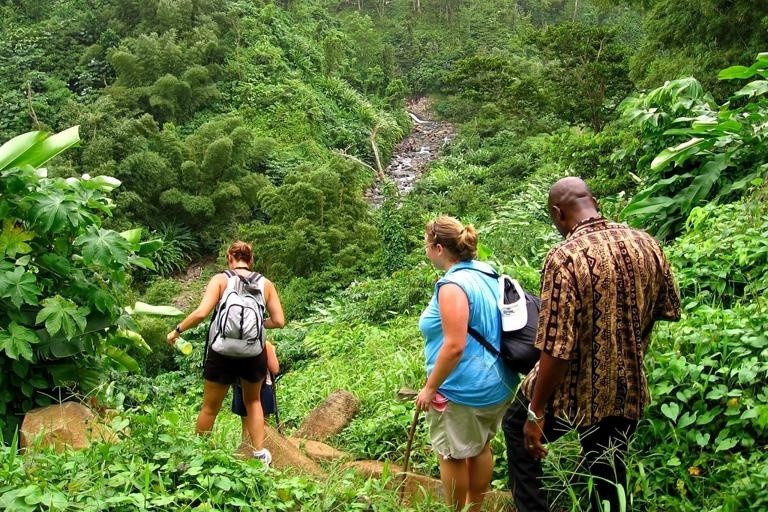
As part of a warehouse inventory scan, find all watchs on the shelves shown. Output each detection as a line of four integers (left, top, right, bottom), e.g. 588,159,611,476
523,402,545,424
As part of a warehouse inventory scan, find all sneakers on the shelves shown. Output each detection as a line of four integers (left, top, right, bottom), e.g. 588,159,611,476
254,447,273,476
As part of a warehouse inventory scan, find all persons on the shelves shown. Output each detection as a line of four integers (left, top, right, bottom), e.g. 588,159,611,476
231,338,282,444
501,175,683,512
165,240,289,469
415,214,522,512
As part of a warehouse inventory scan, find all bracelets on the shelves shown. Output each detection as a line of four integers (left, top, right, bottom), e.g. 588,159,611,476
175,323,182,333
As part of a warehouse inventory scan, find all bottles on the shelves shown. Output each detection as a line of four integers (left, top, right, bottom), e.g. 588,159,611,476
174,336,193,355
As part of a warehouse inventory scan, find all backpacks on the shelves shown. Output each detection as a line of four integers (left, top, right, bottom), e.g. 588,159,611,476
450,261,541,376
208,269,267,358
